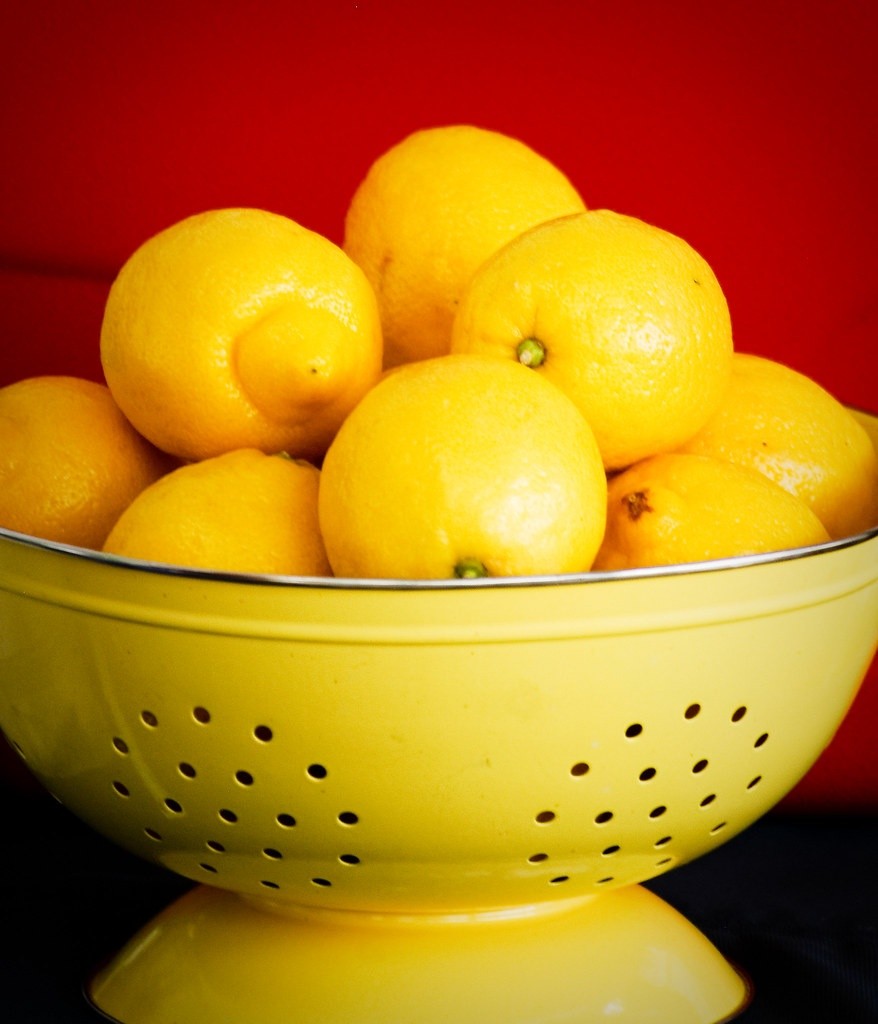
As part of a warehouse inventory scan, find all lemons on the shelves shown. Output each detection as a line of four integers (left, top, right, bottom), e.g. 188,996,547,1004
0,124,878,583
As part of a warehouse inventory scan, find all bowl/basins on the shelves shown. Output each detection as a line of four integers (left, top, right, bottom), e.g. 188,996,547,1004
0,411,878,1024
